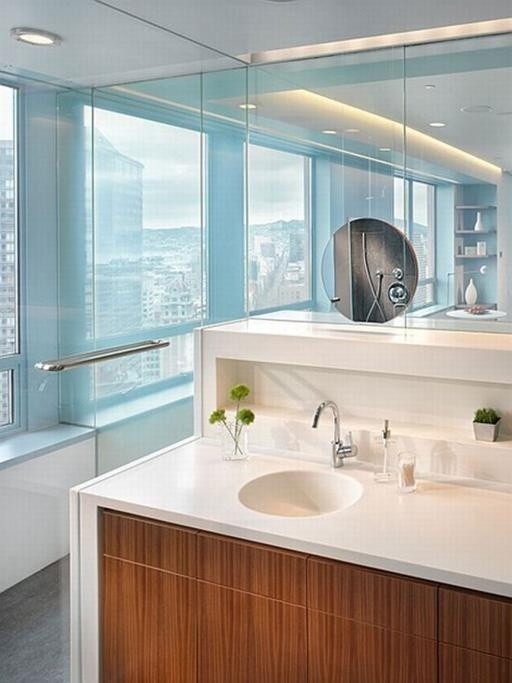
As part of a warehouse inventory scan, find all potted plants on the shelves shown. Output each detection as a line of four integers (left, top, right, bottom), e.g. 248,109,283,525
472,408,502,442
208,383,257,461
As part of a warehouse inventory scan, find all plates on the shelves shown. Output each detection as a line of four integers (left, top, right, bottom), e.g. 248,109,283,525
446,309,508,320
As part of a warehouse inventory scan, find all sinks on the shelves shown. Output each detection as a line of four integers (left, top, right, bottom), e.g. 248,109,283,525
236,467,364,520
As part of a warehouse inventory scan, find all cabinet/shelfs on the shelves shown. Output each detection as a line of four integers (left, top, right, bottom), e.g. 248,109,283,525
95,510,511,681
454,201,498,310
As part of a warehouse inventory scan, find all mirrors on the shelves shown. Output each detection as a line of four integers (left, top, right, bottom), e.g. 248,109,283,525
317,214,421,328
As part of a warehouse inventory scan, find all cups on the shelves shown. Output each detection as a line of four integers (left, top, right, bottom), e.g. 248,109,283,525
477,240,487,255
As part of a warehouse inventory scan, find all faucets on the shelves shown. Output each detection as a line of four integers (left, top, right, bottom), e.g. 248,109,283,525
311,401,360,468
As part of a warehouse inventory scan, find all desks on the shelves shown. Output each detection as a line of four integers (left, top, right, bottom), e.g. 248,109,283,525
445,307,507,321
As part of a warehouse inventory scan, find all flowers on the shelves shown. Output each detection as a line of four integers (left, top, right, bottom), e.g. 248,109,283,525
464,305,488,313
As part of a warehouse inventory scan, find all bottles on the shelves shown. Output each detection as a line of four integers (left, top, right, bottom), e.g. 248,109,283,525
474,211,485,231
465,278,477,307
396,449,417,493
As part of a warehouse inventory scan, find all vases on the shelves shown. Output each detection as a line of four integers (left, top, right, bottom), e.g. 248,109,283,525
474,210,486,231
464,278,479,304
